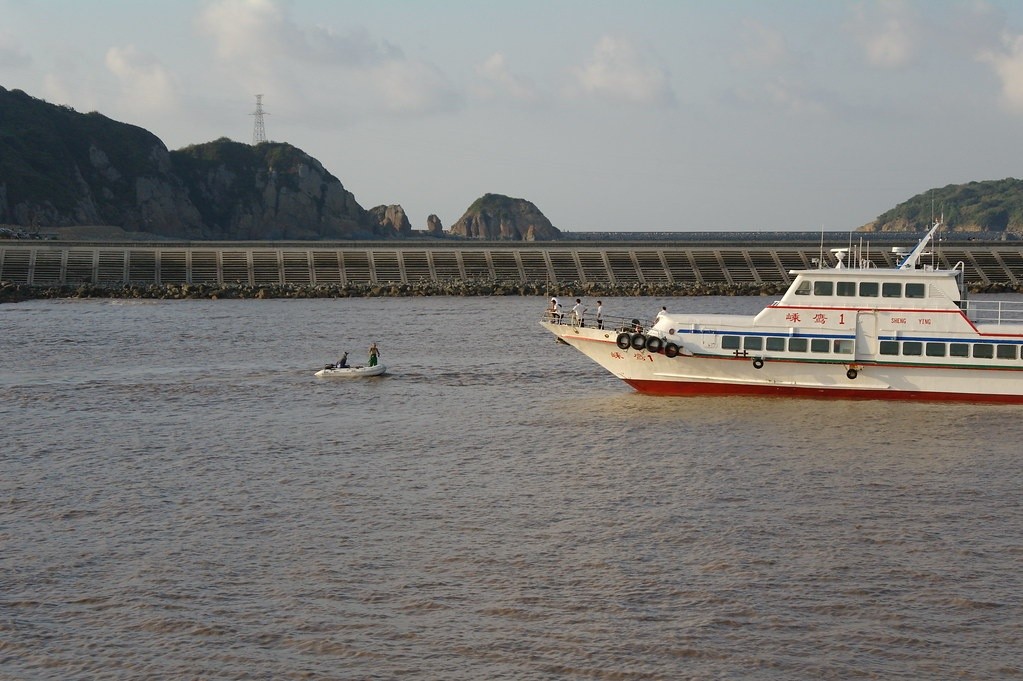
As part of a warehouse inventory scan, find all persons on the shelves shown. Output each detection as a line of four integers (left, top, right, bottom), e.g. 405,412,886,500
548,291,565,327
368,341,381,366
334,350,351,368
597,300,605,330
653,306,668,322
567,298,588,326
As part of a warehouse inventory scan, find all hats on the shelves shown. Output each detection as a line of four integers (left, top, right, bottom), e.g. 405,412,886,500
343,351,349,355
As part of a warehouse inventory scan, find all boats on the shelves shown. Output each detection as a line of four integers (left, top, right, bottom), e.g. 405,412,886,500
541,221,1023,406
315,364,385,376
